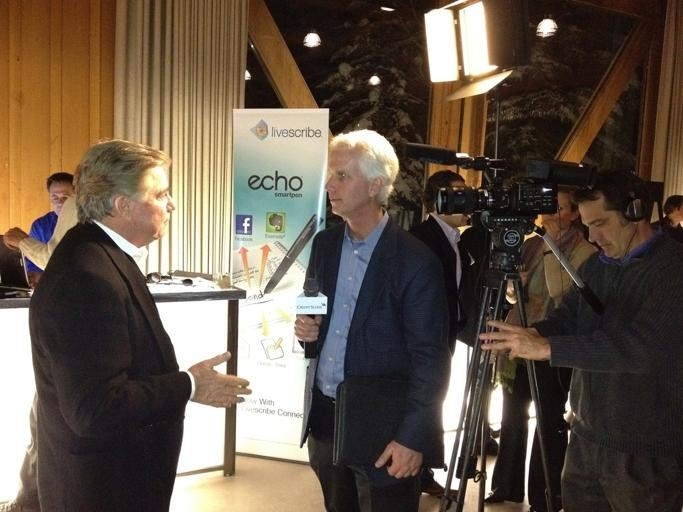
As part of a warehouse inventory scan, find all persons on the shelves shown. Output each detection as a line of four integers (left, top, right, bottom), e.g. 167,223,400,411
4,140,251,511
22,172,77,291
290,126,451,512
412,170,682,512
3,165,79,271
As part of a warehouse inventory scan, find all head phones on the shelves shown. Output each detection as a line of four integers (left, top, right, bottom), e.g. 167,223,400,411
619,168,643,222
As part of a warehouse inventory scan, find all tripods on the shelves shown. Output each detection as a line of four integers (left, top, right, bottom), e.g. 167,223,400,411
437,266,558,512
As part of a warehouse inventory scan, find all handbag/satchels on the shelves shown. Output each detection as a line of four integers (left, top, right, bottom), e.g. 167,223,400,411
332,378,446,467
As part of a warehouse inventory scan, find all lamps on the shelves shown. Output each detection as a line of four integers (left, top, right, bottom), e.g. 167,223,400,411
423,0,530,102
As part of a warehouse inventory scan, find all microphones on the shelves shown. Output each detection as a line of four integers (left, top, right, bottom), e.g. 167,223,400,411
296,280,328,359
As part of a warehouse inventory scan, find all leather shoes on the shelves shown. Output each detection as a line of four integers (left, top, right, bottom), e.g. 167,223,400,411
484,489,525,503
